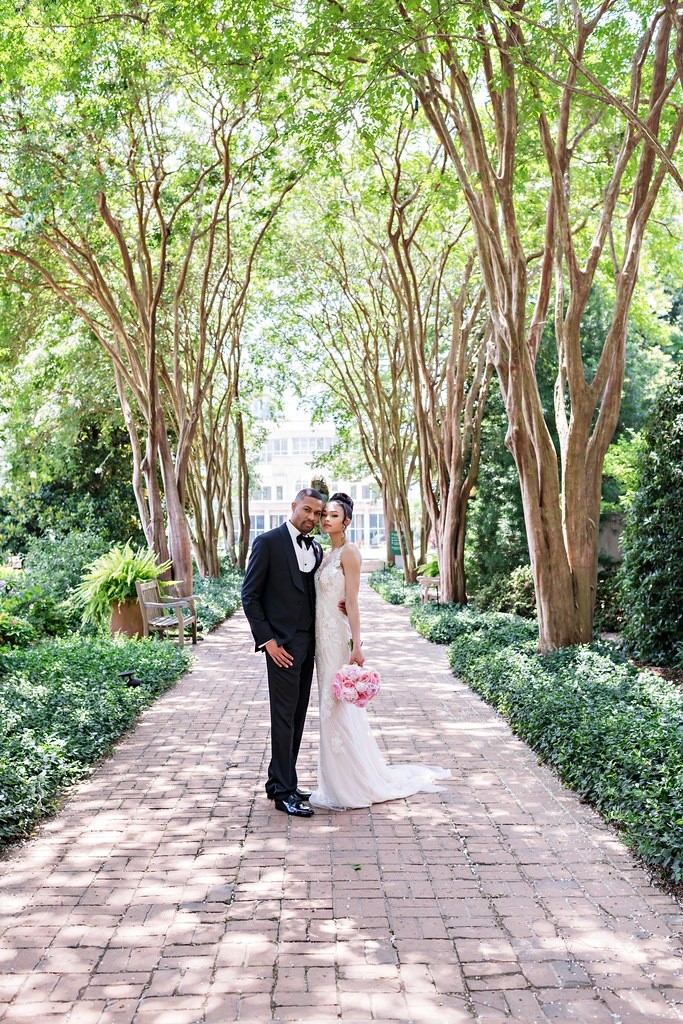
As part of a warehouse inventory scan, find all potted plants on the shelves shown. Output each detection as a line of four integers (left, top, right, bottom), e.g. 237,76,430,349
63,536,183,641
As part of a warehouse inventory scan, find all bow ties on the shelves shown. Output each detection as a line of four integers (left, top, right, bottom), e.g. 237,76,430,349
297,534,314,550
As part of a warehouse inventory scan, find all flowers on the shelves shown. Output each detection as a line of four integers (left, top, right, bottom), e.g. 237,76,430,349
332,638,381,709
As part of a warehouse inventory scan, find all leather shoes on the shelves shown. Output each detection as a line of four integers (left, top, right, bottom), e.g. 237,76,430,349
276,794,314,815
268,788,311,800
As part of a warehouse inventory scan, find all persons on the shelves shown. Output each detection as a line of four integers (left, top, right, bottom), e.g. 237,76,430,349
241,488,348,817
307,493,450,809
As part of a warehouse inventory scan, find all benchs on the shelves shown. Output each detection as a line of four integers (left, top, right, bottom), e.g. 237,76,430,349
416,576,439,605
134,579,202,645
